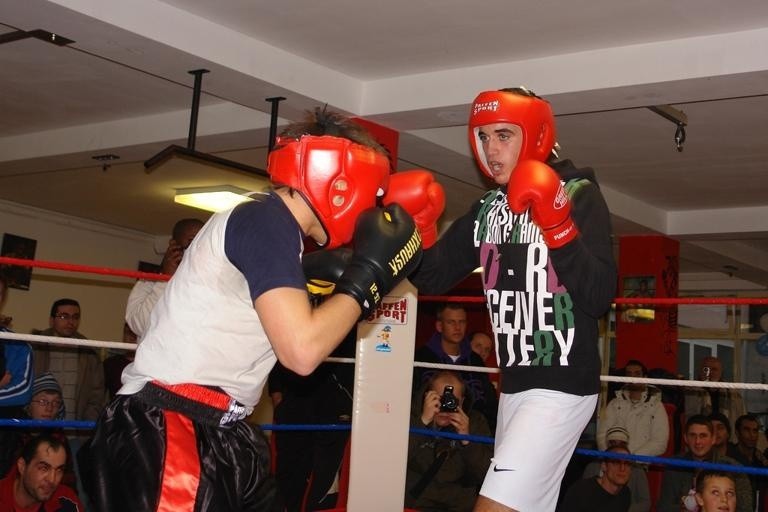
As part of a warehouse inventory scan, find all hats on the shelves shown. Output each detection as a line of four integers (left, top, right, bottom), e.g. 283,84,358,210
31,370,62,397
605,424,628,444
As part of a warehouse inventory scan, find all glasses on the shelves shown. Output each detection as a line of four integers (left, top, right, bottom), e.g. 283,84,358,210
55,313,70,320
32,398,59,407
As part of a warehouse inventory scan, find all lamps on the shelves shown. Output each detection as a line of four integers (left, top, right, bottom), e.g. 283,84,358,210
174,184,254,213
144,67,284,193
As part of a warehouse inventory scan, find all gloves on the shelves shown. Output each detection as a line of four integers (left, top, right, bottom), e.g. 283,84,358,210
381,170,446,251
507,158,579,251
303,247,353,297
332,203,423,322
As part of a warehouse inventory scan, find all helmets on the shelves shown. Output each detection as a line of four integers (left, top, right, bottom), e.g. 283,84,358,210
469,85,555,181
266,129,390,249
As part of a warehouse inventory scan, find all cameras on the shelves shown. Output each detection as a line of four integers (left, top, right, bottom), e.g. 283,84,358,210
438,384,459,411
